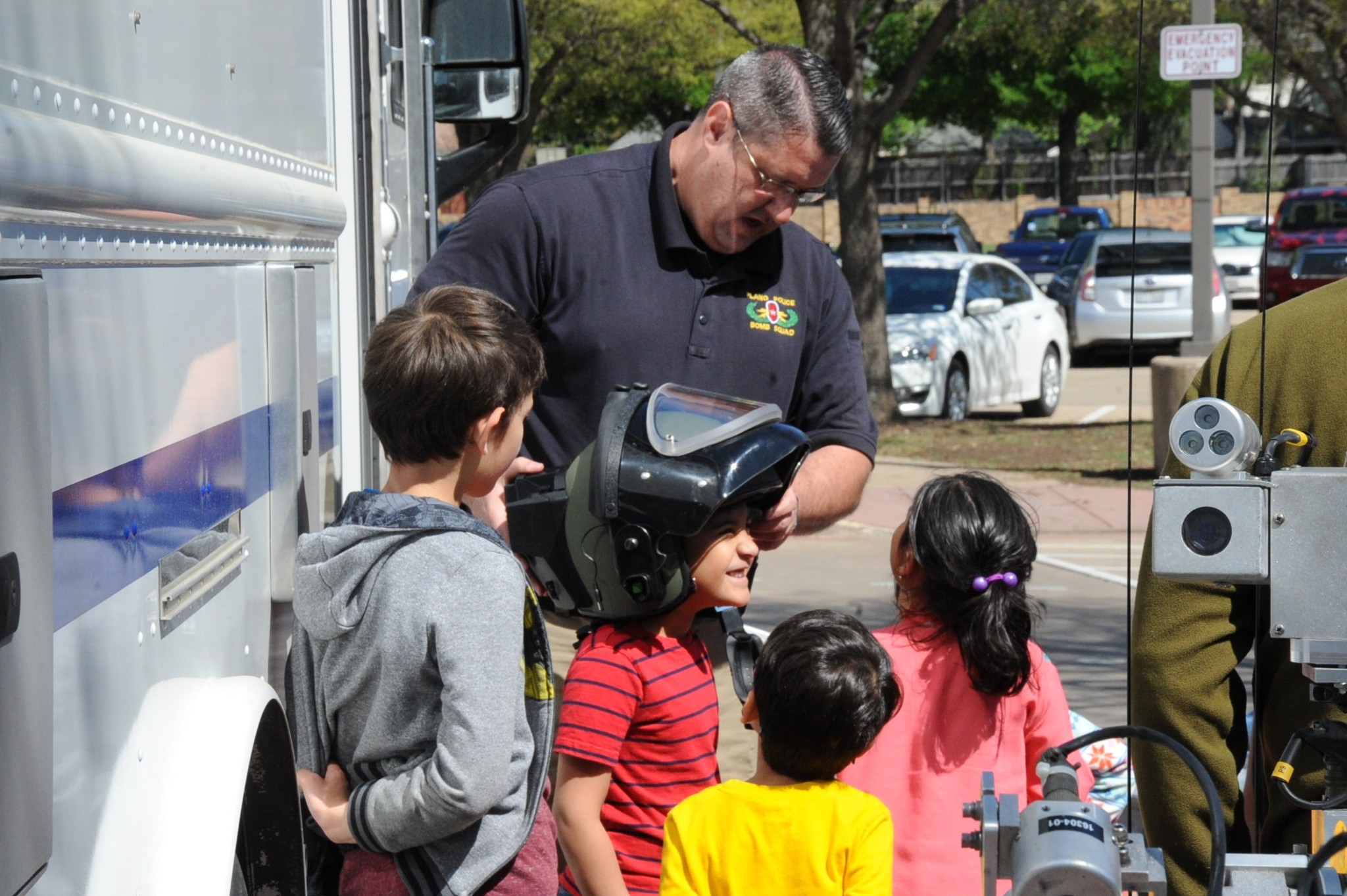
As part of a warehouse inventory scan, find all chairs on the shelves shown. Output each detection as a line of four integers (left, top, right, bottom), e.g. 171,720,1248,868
1295,205,1317,223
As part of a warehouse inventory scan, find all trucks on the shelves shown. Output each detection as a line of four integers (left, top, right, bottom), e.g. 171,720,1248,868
0,1,531,896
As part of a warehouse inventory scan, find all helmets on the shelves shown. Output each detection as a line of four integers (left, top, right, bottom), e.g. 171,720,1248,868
507,378,813,621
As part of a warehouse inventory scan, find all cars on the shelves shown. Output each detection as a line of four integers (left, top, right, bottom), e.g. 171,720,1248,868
836,251,1072,420
1209,214,1274,302
872,212,985,255
1046,226,1231,359
1246,152,1347,311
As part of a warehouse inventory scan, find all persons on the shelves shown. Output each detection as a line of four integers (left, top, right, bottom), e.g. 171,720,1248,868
52,337,270,538
659,609,904,896
404,42,878,681
553,382,813,896
290,284,559,896
834,472,1096,896
1130,276,1347,895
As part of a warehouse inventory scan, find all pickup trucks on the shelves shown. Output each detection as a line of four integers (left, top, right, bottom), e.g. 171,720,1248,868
995,205,1118,289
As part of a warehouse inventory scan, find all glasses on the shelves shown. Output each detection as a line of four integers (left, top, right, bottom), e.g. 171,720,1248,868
728,98,827,207
